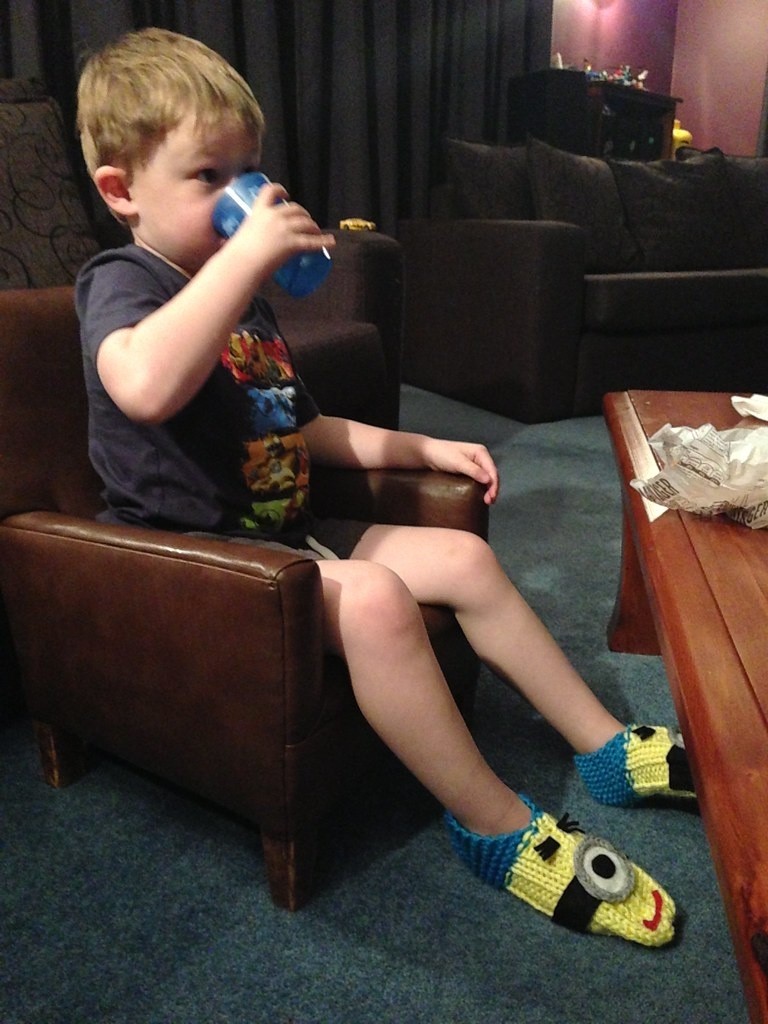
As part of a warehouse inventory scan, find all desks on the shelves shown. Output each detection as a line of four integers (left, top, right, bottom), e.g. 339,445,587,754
507,70,684,163
601,390,768,1024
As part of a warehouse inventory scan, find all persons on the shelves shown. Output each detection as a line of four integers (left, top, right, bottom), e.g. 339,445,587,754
70,25,707,943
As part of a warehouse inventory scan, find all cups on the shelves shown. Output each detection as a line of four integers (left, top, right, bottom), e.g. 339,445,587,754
211,170,331,300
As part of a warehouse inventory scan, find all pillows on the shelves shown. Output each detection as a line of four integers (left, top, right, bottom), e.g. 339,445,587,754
0,93,104,290
429,136,768,273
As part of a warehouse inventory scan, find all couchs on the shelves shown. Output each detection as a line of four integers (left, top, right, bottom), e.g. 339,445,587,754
397,136,768,427
0,74,407,431
0,284,489,911
